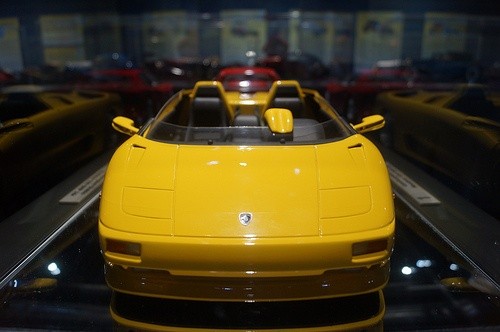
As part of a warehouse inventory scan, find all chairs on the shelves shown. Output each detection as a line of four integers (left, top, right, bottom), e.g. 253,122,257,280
186,97,227,142
273,98,325,144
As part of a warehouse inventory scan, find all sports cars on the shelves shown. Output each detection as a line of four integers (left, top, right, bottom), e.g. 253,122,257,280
97,80,397,303
0,51,500,178
